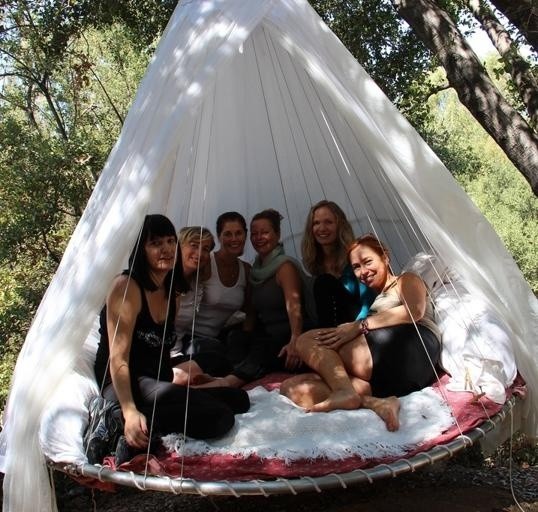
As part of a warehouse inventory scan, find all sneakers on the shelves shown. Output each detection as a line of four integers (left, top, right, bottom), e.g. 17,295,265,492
82,396,142,467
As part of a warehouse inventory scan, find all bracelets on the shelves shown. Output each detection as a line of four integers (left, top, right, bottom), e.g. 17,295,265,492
360,317,369,335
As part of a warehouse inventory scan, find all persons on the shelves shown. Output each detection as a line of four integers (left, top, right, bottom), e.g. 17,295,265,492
169,227,235,388
281,233,443,432
299,200,371,326
220,208,314,374
85,214,250,467
191,212,256,388
